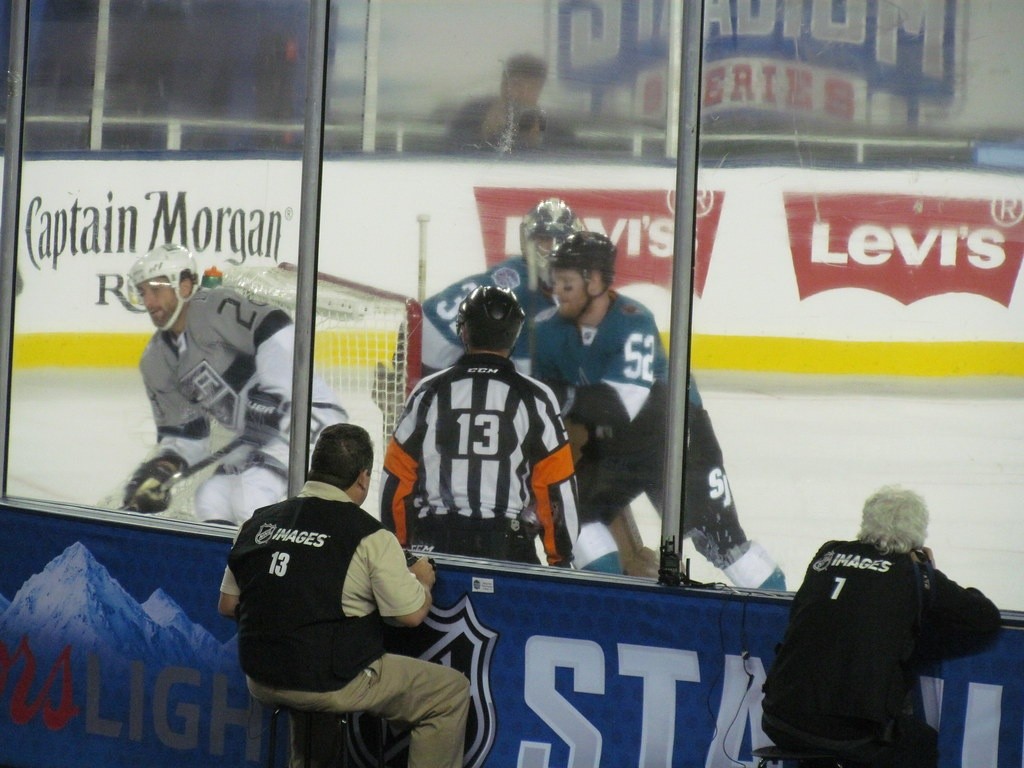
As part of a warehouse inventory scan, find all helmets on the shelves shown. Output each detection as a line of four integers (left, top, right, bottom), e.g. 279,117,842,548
547,230,617,286
128,243,202,303
525,198,575,240
455,284,525,350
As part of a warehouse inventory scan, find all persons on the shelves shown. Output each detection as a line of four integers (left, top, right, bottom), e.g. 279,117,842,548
516,229,786,593
447,54,573,152
378,284,581,568
121,244,350,527
217,425,471,768
392,196,583,394
760,486,1001,768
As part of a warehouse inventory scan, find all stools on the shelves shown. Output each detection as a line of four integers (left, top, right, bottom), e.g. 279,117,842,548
750,746,853,768
269,705,386,768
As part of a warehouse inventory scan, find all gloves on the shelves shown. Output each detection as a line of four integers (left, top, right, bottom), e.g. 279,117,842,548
125,448,191,513
243,384,284,445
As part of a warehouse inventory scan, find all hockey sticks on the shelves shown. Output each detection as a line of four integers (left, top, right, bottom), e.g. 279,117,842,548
117,432,246,512
